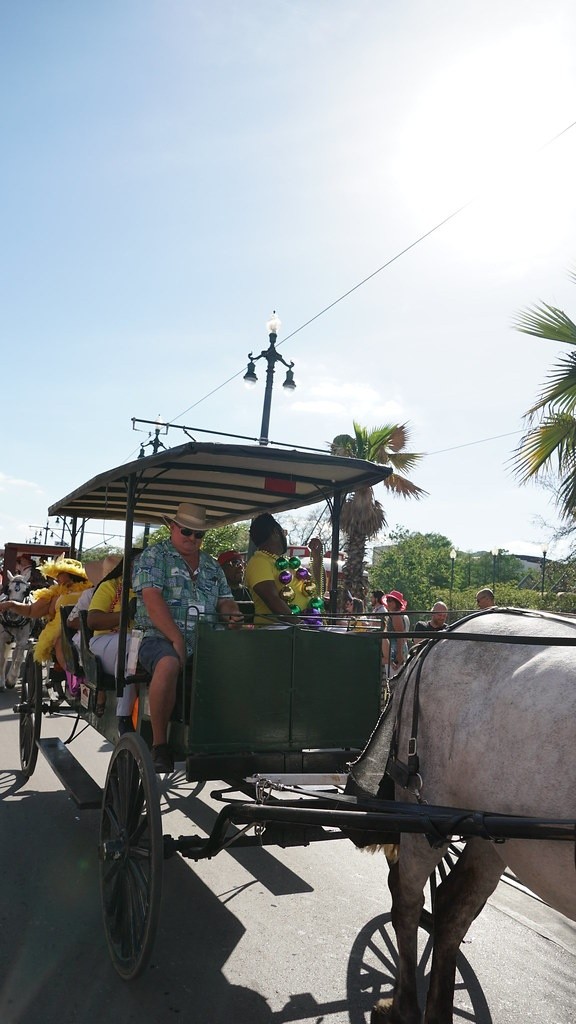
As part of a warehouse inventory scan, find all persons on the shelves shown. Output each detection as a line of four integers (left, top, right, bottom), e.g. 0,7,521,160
0,553,57,643
410,601,450,657
476,588,497,611
215,548,258,625
380,590,410,697
85,544,147,746
348,598,371,632
126,499,245,779
0,557,96,696
336,600,354,627
368,589,388,706
64,554,126,703
242,512,327,626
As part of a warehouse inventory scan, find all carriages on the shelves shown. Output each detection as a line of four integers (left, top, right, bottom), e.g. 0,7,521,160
0,542,77,692
11,417,576,1024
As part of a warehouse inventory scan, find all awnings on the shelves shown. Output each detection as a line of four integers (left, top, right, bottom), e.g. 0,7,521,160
44,439,395,542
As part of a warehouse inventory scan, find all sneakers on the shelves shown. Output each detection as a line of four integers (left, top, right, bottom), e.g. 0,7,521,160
118,716,136,740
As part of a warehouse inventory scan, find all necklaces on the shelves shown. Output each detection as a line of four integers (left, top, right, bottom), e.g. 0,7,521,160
108,578,122,634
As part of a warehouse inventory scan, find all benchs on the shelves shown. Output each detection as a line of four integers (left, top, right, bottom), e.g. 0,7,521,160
59,603,255,691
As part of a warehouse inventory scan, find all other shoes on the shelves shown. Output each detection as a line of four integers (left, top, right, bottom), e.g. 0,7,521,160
94,701,105,717
151,743,173,773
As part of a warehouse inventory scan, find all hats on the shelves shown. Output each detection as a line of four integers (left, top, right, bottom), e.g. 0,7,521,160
323,591,331,600
218,551,243,566
34,552,88,581
161,503,216,536
16,553,33,566
381,590,407,612
85,556,121,588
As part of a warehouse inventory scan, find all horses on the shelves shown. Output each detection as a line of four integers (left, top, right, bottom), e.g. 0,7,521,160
355,605,576,1024
0,568,37,694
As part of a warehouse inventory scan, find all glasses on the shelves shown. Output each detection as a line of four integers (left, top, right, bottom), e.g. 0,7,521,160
175,525,205,539
279,530,287,536
386,599,395,603
477,597,487,603
227,559,248,570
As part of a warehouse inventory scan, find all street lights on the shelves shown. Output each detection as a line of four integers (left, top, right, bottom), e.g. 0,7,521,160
243,309,297,561
449,548,457,623
541,542,548,601
490,546,498,593
25,514,74,545
137,429,174,547
